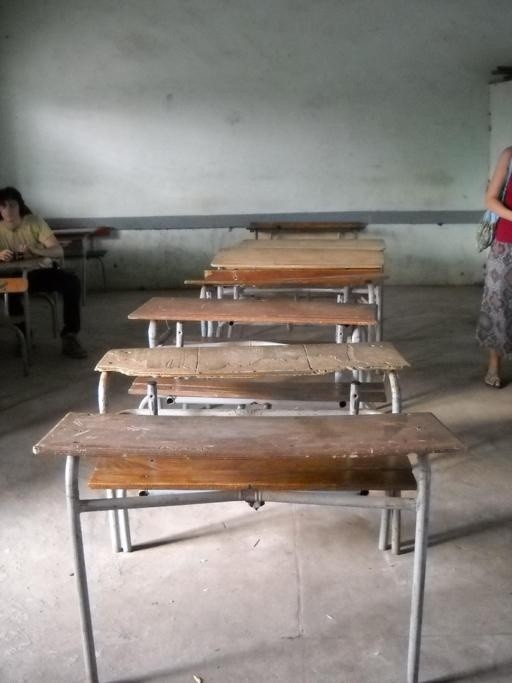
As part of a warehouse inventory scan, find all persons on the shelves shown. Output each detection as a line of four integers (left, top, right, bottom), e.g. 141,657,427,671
1,184,90,359
472,144,512,387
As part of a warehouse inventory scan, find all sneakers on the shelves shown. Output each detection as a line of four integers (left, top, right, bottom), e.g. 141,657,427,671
15,334,36,357
60,335,89,359
484,365,505,388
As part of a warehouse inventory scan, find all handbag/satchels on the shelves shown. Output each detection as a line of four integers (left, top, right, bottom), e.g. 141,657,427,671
475,208,499,254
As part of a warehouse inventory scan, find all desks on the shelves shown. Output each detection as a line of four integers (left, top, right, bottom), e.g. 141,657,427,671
93,342,411,554
32,412,469,682
127,222,388,411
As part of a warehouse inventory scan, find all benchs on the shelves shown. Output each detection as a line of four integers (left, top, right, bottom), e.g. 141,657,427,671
3,226,115,373
87,453,416,554
